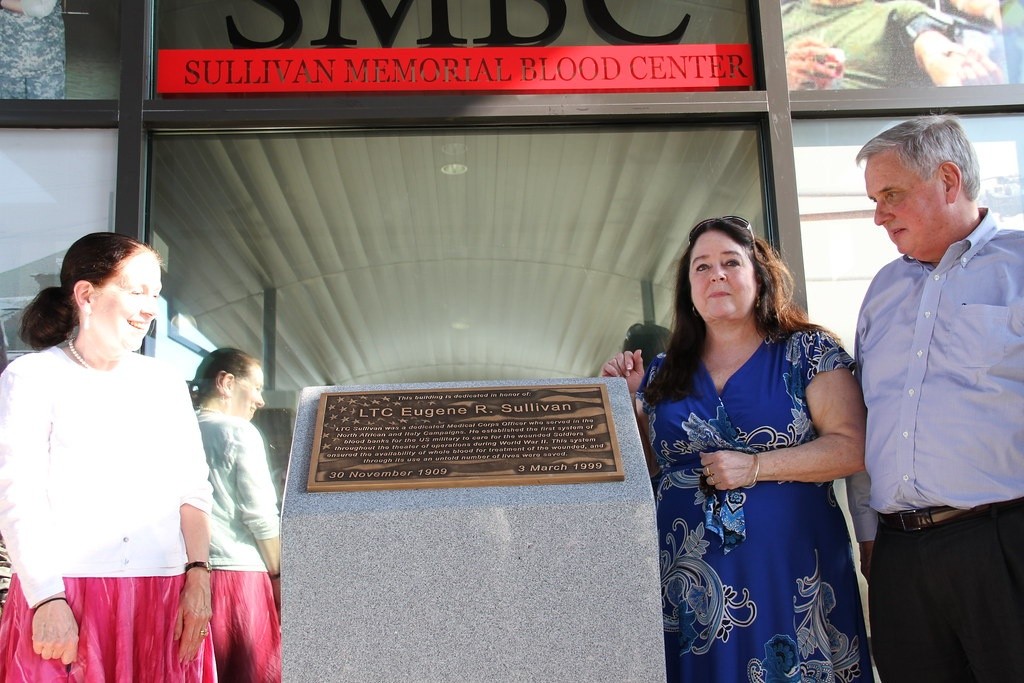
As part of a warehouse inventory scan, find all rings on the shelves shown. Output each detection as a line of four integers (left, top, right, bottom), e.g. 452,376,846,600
710,476,717,484
706,465,712,476
201,629,207,636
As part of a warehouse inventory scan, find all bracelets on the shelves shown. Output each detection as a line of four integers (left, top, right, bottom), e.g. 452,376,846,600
270,573,280,579
748,453,759,487
34,597,68,615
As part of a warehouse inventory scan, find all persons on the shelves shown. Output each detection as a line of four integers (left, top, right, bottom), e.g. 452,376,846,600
780,0,1001,91
0,231,219,683
188,346,281,683
601,217,875,683
623,323,676,373
846,118,1024,683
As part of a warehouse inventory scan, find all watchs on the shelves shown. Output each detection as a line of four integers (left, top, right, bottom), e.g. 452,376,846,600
185,561,212,573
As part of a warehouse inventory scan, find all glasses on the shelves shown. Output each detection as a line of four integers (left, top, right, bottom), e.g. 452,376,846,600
688,215,756,249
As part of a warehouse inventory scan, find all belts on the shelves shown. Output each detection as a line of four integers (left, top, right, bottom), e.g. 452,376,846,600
877,501,996,532
